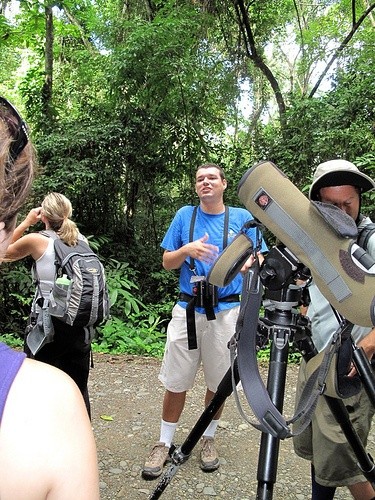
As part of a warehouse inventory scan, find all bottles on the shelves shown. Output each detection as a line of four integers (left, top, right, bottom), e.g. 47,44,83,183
56,274,72,291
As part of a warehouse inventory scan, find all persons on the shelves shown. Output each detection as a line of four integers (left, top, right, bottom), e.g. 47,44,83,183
5,192,91,418
0,96,100,500
240,160,375,500
142,163,269,476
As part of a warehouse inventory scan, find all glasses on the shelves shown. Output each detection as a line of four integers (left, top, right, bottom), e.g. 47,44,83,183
0,97,29,174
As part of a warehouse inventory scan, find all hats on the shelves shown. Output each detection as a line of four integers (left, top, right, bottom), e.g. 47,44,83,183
309,160,375,200
27,309,54,356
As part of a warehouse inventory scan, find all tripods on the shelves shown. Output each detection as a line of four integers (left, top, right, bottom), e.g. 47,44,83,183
150,245,375,500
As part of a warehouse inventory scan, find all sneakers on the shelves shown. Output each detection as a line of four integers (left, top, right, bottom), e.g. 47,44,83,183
143,442,170,477
200,436,219,471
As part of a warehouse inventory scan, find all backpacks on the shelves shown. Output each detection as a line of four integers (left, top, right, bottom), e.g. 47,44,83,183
42,230,109,327
